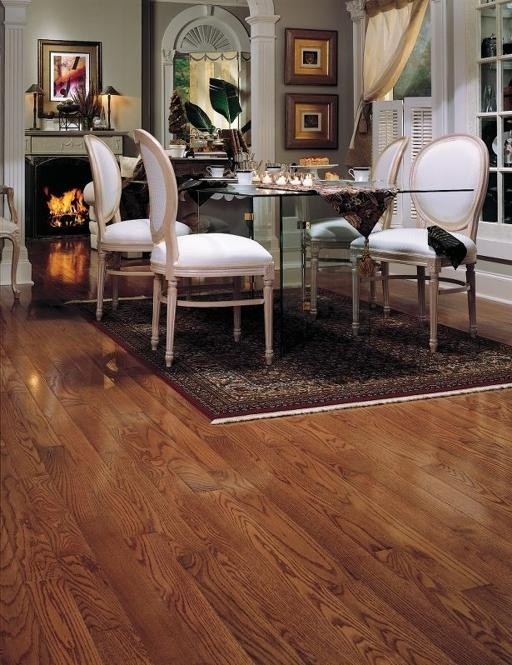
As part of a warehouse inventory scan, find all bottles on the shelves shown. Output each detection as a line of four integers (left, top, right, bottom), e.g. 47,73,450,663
482,80,496,111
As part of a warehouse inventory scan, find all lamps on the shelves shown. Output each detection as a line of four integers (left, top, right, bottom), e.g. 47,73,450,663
99,85,120,129
26,83,46,129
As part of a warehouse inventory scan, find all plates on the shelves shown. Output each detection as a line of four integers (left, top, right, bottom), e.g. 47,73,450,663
228,183,257,187
320,179,343,182
203,175,230,179
345,179,374,183
289,163,339,169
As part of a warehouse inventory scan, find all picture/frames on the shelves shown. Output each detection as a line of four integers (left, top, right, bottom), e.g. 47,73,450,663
284,27,338,86
38,38,102,119
285,92,340,149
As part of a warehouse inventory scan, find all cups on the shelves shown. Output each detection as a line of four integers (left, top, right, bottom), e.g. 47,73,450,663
206,165,226,176
246,160,314,187
349,166,371,182
236,170,253,184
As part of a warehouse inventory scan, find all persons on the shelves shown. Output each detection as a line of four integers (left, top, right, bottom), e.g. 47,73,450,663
481,82,512,224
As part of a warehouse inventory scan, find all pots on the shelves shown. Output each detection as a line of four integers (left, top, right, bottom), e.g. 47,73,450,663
481,32,503,57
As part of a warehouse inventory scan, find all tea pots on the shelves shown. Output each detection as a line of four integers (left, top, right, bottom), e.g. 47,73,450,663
234,148,255,170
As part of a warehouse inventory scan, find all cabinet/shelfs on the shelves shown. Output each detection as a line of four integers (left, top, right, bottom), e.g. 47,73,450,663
453,0,512,262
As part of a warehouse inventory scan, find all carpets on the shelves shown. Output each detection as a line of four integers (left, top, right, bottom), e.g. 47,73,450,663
68,287,509,426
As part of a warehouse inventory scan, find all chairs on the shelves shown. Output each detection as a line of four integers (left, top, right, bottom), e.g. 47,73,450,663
82,146,185,251
133,128,275,366
350,135,489,354
0,186,23,300
300,137,410,319
85,134,191,321
